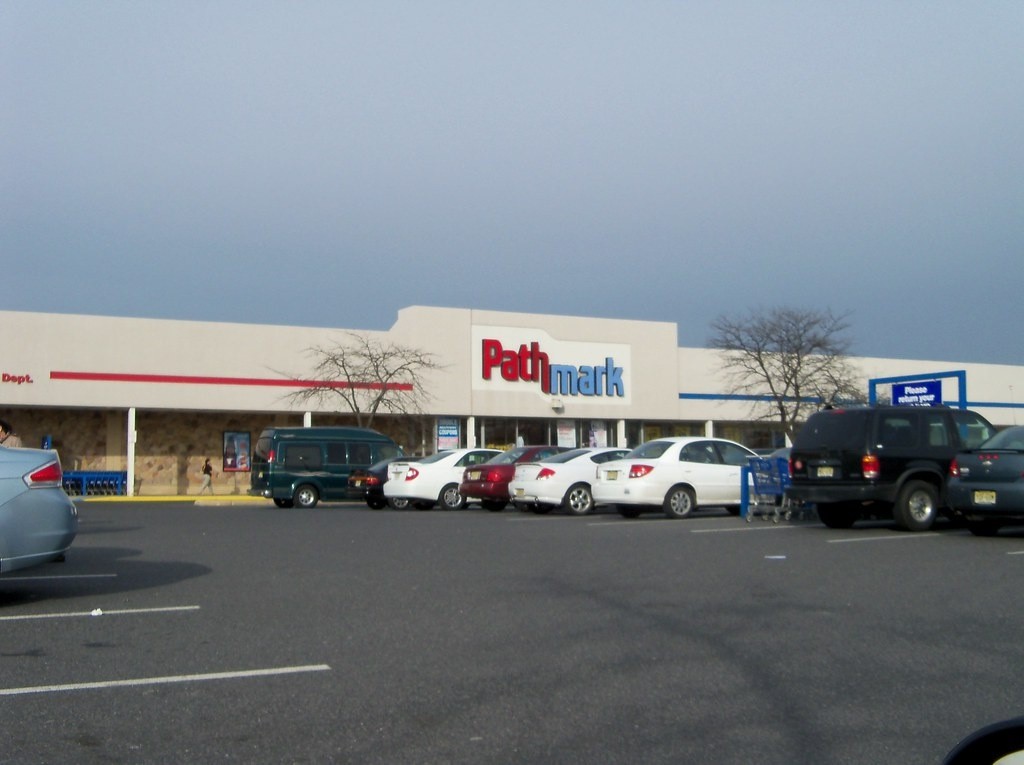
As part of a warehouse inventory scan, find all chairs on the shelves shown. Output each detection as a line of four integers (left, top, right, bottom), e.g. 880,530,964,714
885,423,920,446
688,450,719,463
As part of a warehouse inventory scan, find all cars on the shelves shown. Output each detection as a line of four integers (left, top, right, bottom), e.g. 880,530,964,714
591,436,790,521
0,444,78,573
381,449,506,512
347,456,425,512
507,447,635,516
947,424,1024,537
458,445,576,513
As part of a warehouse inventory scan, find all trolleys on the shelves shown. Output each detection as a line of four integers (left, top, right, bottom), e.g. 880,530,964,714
741,454,807,524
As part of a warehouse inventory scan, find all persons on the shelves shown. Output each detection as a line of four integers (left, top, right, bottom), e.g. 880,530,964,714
197,459,214,495
0,420,22,448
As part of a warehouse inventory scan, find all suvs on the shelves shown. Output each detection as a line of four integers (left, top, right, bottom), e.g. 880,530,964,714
785,405,999,534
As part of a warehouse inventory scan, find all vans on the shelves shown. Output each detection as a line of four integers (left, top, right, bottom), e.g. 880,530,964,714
244,426,412,511
